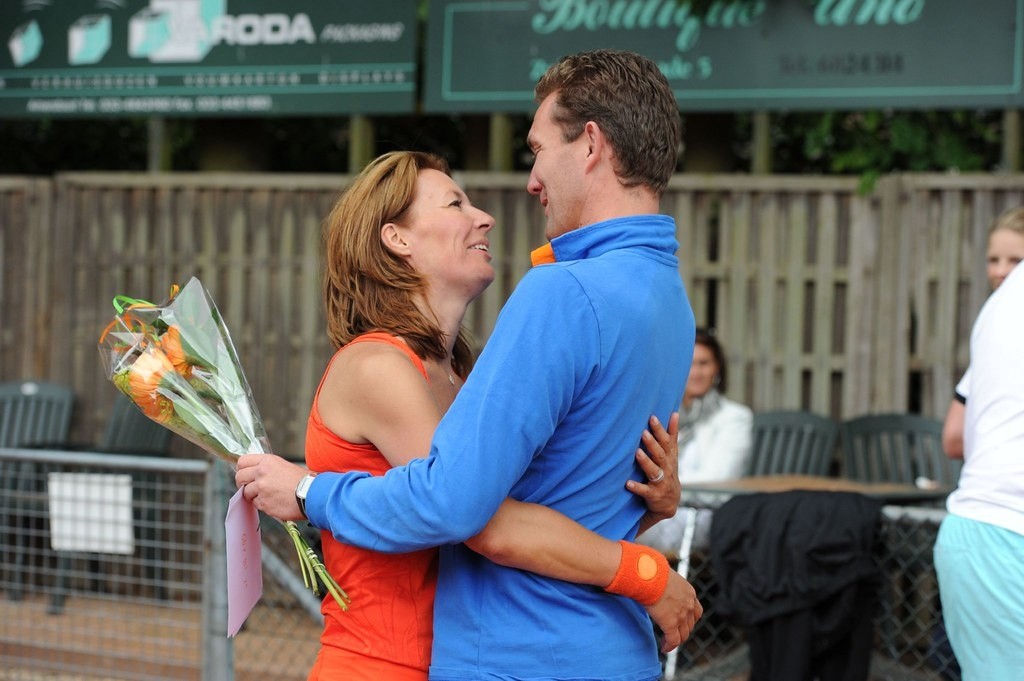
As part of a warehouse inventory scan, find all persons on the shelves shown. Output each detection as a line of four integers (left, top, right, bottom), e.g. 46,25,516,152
932,257,1024,681
634,325,753,555
236,51,695,679
304,149,703,681
984,203,1024,290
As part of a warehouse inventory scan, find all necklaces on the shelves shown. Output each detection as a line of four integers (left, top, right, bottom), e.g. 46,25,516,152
445,366,455,385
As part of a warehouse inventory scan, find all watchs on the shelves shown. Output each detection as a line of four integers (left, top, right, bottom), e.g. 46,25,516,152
295,471,320,527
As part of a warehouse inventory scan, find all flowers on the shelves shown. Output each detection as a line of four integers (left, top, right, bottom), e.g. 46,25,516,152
103,296,355,613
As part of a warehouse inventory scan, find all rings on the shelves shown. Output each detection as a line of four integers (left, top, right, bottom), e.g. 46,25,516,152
649,468,664,482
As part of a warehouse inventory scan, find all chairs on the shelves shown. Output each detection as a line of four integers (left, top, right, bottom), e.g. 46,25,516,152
51,393,174,613
743,407,843,479
0,385,81,591
837,412,960,492
